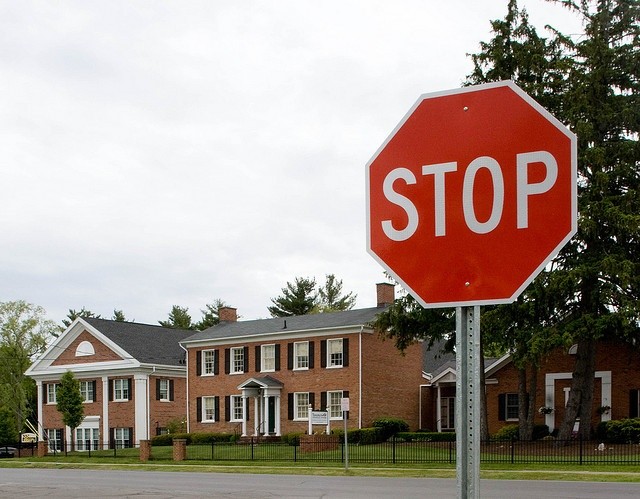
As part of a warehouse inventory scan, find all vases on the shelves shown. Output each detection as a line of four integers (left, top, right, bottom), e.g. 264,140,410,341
545,409,553,414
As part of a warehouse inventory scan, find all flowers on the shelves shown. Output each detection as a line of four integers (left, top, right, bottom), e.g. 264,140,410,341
539,407,555,416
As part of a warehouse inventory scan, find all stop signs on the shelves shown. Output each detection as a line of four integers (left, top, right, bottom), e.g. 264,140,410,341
365,79,577,310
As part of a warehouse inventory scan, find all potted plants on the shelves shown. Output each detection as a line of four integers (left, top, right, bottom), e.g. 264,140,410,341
597,406,610,415
